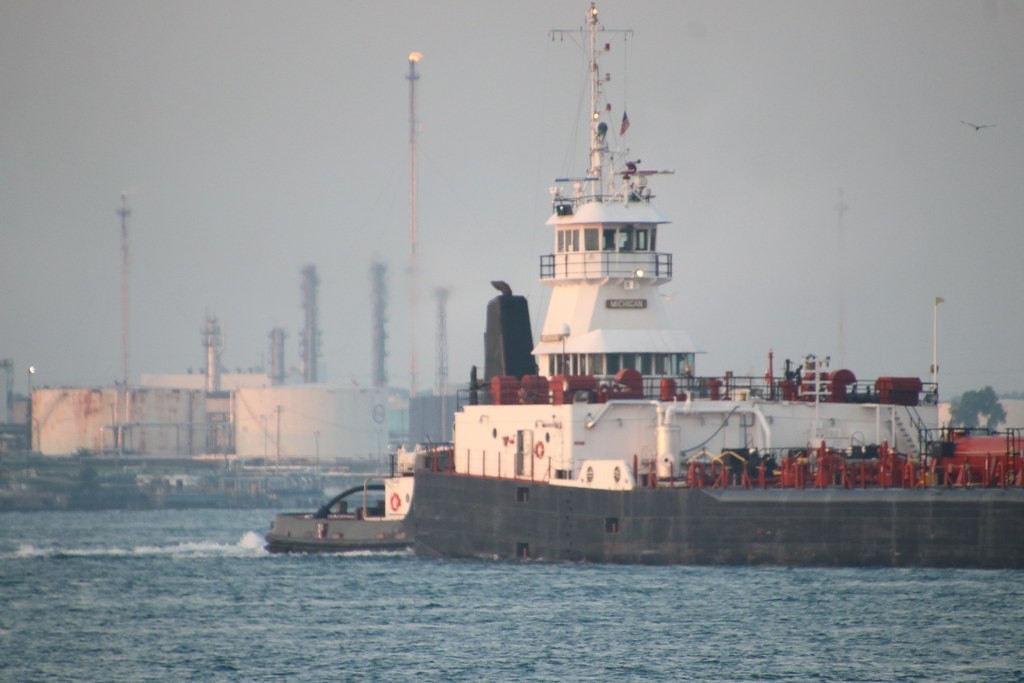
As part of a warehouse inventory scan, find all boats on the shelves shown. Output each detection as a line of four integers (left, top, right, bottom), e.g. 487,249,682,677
381,0,1023,574
262,475,415,552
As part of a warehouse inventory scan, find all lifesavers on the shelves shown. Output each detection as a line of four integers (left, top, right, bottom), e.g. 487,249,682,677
535,441,544,459
389,493,401,511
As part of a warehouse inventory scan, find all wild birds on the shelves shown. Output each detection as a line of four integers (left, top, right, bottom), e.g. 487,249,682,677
960,120,998,132
